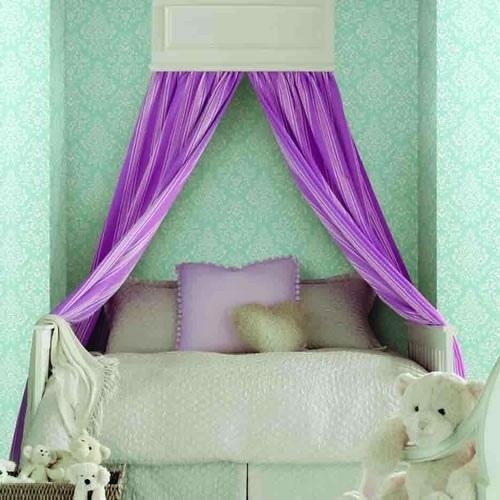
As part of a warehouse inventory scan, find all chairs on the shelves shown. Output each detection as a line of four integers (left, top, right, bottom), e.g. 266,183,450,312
384,344,500,499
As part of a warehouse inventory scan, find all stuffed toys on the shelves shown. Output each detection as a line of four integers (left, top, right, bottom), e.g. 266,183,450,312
68,463,111,500
18,443,63,483
50,429,111,482
0,459,17,483
363,369,485,500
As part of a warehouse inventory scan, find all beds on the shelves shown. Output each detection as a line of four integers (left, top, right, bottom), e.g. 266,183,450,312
32,0,454,498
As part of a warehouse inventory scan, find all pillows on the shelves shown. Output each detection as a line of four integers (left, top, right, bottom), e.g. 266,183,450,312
174,255,301,355
98,274,182,354
297,271,389,353
237,302,311,351
